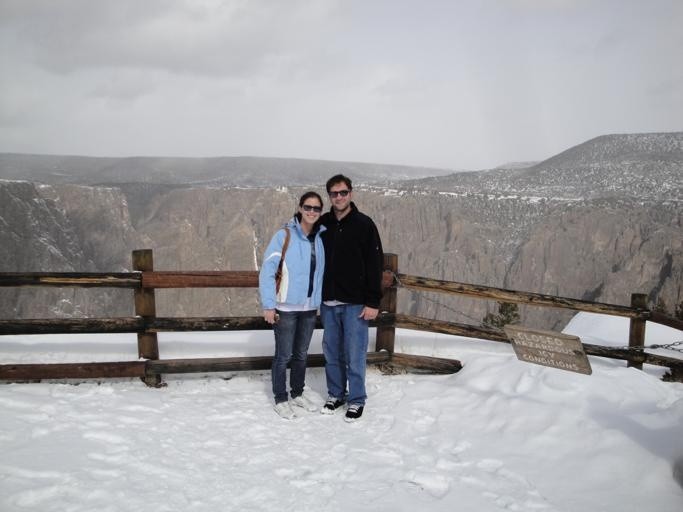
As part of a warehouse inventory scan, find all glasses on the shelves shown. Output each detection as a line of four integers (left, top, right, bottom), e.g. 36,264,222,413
302,205,322,213
328,190,350,198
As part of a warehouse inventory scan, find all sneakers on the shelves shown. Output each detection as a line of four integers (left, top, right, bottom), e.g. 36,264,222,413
273,401,297,421
290,394,317,412
345,403,363,418
323,394,347,410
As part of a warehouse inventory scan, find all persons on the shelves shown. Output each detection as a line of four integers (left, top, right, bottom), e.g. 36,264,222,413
313,175,385,421
259,191,328,420
